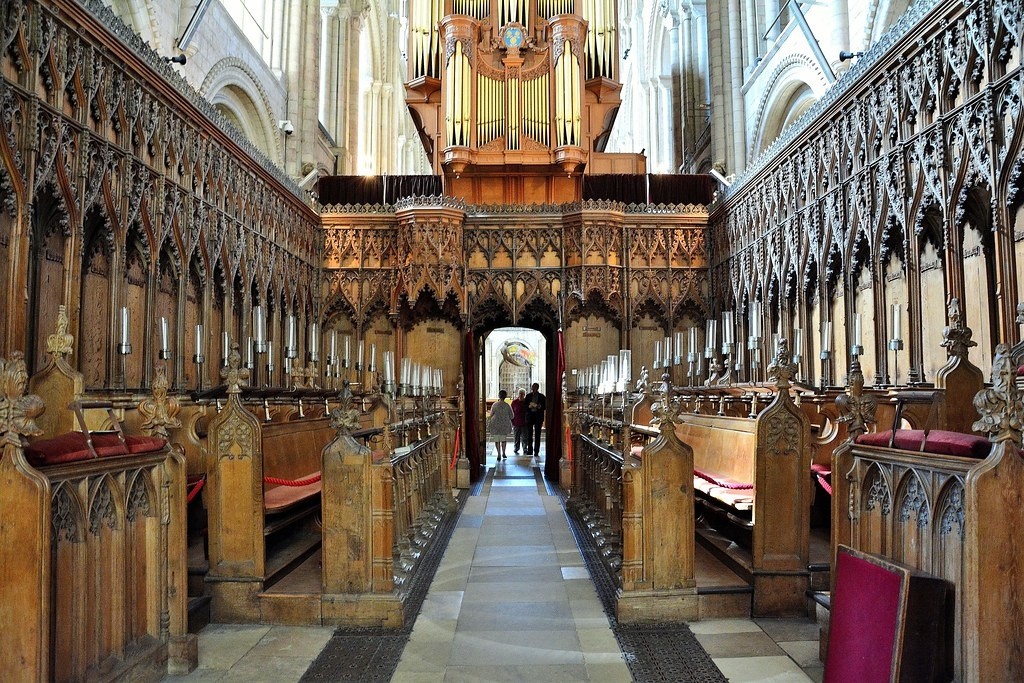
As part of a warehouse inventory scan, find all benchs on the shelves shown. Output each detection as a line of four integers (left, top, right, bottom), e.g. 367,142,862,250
633,383,895,577
164,398,405,586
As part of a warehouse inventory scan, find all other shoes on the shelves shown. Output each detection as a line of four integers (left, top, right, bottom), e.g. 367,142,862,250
534,452,538,456
513,450,518,452
502,455,507,458
524,450,527,453
497,455,501,461
527,451,533,455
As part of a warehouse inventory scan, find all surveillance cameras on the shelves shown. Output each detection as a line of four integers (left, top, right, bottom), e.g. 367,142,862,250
284,124,293,135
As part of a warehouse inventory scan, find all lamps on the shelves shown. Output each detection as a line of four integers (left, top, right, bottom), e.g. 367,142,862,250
292,166,320,189
839,50,863,63
708,169,736,188
165,53,187,65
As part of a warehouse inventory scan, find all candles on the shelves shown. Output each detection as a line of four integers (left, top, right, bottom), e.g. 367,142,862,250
576,299,832,395
195,324,200,355
122,306,128,344
894,303,901,340
162,316,168,351
221,305,444,389
855,313,863,347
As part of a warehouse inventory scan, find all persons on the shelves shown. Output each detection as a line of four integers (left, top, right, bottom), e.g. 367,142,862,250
489,390,514,461
511,389,528,453
524,383,545,456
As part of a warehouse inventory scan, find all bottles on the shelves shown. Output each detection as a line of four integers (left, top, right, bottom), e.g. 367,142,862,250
640,148,645,156
